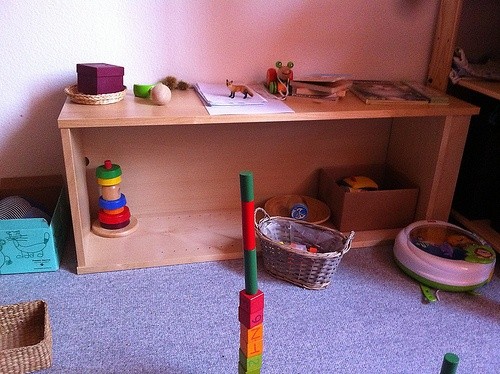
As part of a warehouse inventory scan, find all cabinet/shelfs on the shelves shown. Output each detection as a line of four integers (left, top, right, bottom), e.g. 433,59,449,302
451,75,500,253
57,81,481,276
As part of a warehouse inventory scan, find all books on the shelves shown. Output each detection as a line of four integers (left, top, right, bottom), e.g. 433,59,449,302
349,80,451,104
292,86,346,96
292,74,353,94
286,95,339,104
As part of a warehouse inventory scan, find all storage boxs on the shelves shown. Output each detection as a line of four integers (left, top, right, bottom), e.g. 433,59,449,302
76,62,125,94
0,174,71,274
318,162,419,232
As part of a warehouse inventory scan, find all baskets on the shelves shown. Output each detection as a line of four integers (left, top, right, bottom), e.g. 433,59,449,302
0,300,51,374
254,206,354,290
64,83,127,105
